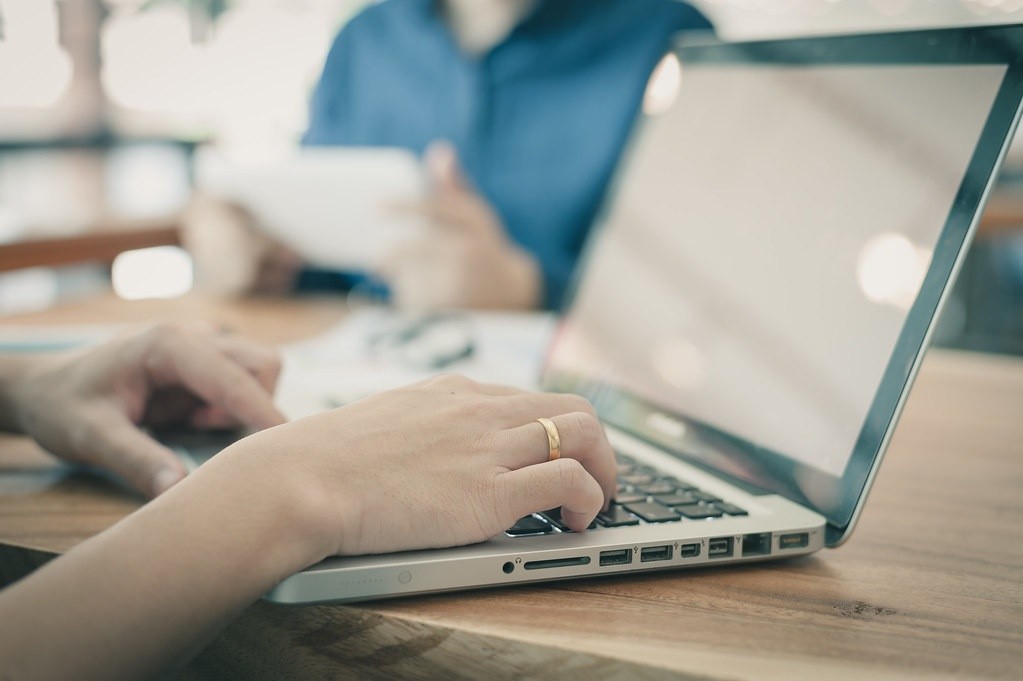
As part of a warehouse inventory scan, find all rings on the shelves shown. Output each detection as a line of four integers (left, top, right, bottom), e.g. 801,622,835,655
537,417,561,461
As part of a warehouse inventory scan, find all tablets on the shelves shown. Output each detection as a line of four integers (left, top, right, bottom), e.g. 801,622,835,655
192,141,433,273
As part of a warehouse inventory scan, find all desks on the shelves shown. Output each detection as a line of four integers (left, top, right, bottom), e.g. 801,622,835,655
0,282,1023,681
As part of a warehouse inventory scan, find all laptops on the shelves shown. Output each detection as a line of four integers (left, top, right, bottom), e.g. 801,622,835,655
164,23,1023,606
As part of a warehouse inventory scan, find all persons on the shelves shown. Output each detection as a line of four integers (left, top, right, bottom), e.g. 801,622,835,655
177,1,714,313
0,320,618,680
